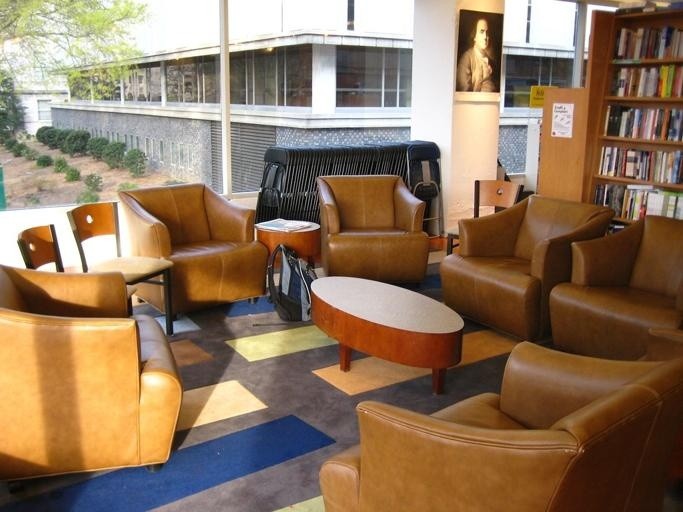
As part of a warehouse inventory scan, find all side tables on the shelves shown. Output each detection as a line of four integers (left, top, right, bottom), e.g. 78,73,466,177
257,220,321,269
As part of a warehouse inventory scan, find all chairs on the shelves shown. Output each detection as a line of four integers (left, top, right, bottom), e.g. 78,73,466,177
118,181,269,321
67,202,174,335
319,342,683,512
447,179,525,255
439,194,616,341
549,216,683,360
17,224,137,314
317,174,430,288
0,263,184,493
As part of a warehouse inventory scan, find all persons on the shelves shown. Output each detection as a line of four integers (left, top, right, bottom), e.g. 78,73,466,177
458,14,501,94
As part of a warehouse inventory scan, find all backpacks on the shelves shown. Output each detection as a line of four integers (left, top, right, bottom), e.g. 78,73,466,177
266,243,320,322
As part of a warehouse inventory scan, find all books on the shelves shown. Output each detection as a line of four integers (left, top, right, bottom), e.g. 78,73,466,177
255,217,312,233
593,1,682,232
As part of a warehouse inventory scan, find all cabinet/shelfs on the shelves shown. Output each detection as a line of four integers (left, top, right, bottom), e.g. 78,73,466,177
580,9,683,227
